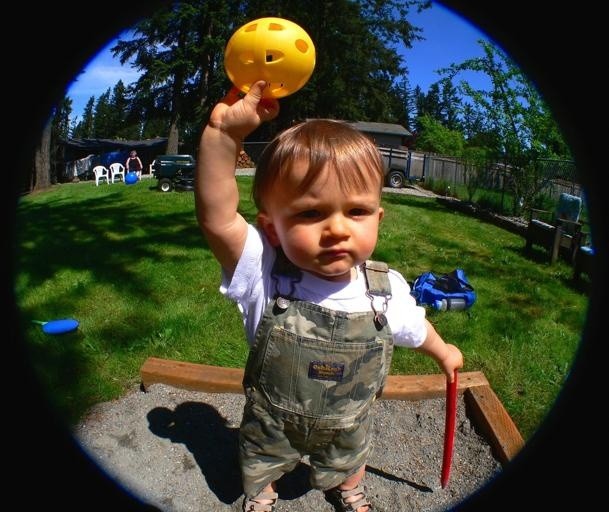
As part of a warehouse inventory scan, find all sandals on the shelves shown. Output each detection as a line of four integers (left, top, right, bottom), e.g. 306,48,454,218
333,485,373,511
243,491,278,511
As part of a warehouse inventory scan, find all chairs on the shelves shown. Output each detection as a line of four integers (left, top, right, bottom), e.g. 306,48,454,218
526,193,584,268
568,230,594,279
150,160,156,179
127,168,142,181
93,166,110,187
109,162,126,184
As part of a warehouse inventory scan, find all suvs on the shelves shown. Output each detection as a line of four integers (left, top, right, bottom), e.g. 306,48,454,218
154,154,196,193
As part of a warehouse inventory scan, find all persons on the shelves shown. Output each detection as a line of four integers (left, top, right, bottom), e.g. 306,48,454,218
191,74,467,512
124,150,144,177
